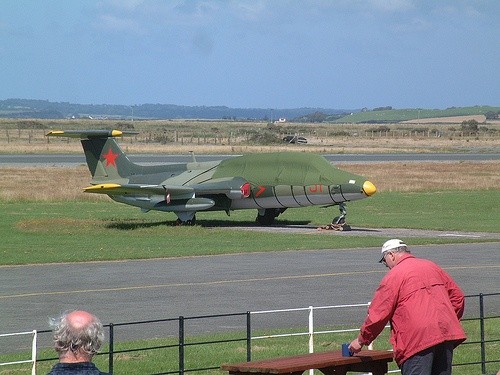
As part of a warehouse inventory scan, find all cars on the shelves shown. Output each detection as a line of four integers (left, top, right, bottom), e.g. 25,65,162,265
284,135,307,143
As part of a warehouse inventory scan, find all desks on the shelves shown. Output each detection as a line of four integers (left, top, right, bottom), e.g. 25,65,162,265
222,350,394,375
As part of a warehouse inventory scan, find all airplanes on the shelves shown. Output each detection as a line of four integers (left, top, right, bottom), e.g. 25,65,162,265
46,130,378,232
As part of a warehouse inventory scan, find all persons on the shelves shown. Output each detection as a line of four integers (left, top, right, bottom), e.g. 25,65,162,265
45,310,110,375
347,239,467,375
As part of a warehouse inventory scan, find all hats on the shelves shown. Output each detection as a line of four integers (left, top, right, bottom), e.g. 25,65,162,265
377,239,407,263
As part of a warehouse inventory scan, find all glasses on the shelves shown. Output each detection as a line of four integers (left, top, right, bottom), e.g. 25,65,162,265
382,250,394,262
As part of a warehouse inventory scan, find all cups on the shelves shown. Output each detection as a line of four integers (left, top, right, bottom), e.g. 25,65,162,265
342,343,354,357
